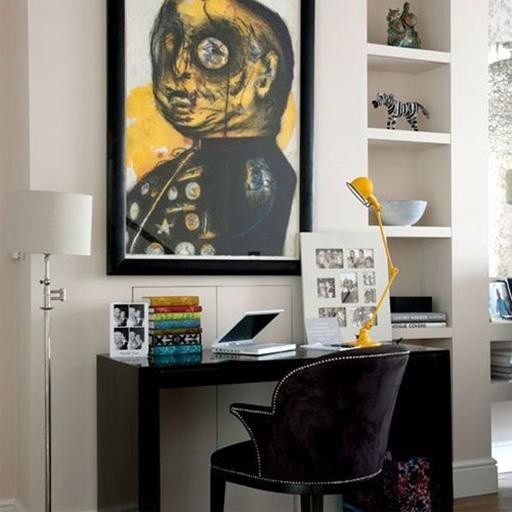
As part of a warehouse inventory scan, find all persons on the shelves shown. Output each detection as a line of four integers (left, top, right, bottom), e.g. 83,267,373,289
317,248,376,327
114,304,144,349
495,288,510,319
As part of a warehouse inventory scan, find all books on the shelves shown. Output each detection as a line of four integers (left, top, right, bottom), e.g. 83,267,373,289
141,296,203,356
148,354,202,365
491,349,512,379
391,312,446,327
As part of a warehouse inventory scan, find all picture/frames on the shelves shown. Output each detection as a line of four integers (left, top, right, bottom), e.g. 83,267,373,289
489,278,512,322
105,0,315,276
109,301,150,359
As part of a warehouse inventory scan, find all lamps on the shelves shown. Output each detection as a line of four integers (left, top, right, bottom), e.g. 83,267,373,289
4,190,93,512
342,177,400,349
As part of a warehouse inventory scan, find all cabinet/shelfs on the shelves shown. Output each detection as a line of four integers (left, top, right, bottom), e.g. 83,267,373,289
366,0,453,341
488,322,512,402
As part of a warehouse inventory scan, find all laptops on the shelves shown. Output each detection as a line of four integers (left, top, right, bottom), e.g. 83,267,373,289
210,310,297,357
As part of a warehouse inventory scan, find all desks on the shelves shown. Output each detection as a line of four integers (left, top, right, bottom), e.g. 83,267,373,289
96,343,454,512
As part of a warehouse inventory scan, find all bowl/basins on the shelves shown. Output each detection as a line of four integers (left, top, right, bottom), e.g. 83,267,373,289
381,201,428,226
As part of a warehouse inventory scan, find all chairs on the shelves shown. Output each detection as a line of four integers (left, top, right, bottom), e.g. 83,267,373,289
209,343,410,512
